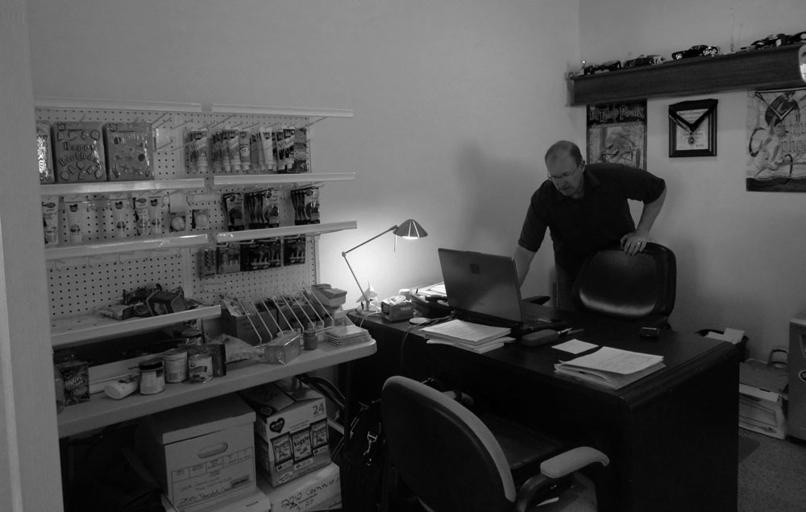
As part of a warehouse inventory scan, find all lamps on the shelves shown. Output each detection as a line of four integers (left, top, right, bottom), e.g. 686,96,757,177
341,219,427,317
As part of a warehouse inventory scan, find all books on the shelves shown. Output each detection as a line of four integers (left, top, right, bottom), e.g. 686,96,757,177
420,317,513,355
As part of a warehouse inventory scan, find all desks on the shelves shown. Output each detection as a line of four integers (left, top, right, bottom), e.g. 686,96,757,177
346,298,740,512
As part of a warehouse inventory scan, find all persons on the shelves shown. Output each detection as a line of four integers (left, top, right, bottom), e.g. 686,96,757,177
513,141,666,307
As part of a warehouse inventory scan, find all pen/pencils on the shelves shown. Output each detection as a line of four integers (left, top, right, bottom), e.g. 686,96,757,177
566,328,584,336
557,327,573,334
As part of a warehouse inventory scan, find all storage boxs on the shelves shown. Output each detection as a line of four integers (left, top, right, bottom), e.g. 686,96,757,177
160,488,270,512
258,462,342,512
150,393,258,506
242,376,332,487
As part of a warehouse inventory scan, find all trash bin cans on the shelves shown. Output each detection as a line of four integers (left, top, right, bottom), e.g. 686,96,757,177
694,329,750,363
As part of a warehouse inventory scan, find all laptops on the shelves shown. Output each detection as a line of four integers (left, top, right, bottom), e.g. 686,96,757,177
438,248,555,329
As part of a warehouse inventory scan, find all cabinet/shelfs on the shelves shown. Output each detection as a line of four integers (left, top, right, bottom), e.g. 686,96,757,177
33,101,378,442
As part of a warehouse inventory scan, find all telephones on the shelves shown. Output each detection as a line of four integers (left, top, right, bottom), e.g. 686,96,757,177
411,293,451,319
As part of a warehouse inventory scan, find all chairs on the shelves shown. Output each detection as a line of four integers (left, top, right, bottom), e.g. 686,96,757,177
381,376,610,512
570,241,678,330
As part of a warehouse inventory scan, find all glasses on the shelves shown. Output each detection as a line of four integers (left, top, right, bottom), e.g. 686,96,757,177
546,165,579,180
